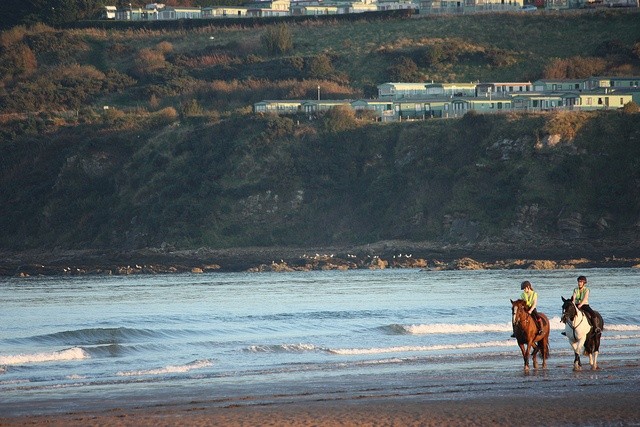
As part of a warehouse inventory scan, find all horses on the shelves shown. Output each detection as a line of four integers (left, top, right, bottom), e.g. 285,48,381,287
561,295,604,371
509,298,551,371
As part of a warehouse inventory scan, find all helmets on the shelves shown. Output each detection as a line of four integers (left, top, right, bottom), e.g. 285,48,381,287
520,281,531,290
577,275,587,289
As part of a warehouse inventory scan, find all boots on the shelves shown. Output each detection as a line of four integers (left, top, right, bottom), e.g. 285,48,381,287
593,317,600,333
560,332,566,336
537,319,543,333
511,334,516,338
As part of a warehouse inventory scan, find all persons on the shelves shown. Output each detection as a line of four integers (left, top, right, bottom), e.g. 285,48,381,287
572,274,600,332
521,280,543,333
